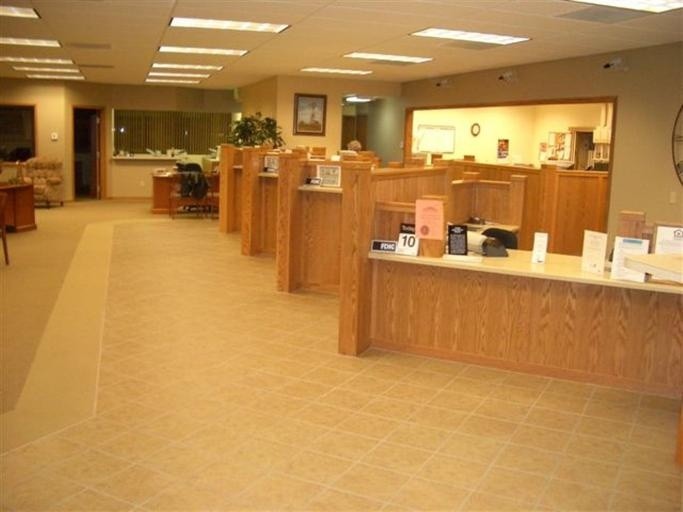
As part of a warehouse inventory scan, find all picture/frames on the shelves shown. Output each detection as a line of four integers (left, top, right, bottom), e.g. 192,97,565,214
497,138,509,159
292,92,328,137
316,164,341,187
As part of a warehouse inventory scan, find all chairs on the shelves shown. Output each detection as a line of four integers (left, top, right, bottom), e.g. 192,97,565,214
16,156,65,208
0,191,10,265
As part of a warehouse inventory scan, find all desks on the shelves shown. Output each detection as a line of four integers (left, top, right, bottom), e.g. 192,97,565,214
366,244,683,398
149,172,219,214
0,181,37,233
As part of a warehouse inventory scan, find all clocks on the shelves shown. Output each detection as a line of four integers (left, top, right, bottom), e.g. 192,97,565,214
471,122,480,136
